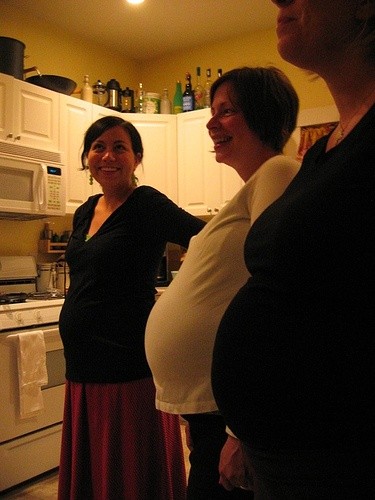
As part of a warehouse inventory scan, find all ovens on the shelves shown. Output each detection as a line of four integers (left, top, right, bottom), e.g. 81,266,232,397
0,324,67,492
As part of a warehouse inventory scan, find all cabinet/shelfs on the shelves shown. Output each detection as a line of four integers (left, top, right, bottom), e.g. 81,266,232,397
0,74,59,152
177,108,245,221
60,94,178,213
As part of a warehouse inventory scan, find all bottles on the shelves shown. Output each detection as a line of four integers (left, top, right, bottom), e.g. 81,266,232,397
193,67,203,110
204,68,213,108
172,79,183,114
160,88,172,114
81,75,93,103
120,87,134,113
43,223,72,250
182,72,193,112
55,266,70,297
36,262,56,292
134,82,145,113
106,79,121,112
218,69,222,79
93,80,107,107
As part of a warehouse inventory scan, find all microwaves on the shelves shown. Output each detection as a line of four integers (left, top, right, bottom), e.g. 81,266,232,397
0,152,67,222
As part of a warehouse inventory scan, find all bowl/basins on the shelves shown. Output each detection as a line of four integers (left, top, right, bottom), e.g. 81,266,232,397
26,74,78,96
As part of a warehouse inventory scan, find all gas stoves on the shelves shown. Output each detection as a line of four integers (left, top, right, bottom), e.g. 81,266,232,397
0,255,66,332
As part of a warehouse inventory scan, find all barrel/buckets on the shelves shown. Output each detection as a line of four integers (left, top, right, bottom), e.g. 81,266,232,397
0,36,26,80
145,92,161,114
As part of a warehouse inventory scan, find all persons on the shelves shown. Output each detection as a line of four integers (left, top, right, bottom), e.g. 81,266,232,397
142,67,303,500
209,0,374,500
58,116,209,500
